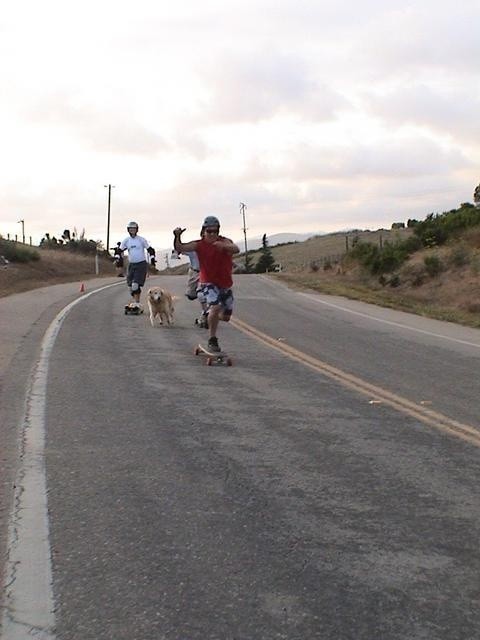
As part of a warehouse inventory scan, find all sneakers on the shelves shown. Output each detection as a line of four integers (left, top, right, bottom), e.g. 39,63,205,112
208,336,221,352
202,309,209,319
133,303,141,308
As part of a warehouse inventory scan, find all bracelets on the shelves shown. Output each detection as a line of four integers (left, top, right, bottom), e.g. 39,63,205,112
150,255,154,259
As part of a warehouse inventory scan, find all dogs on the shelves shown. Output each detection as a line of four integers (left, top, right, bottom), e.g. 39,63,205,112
146,286,176,330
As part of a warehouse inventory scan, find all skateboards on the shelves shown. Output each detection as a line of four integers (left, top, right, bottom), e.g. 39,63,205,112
195,343,232,367
194,318,209,329
123,305,145,315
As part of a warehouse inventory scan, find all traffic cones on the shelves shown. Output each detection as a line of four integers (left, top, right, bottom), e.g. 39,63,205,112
80,284,85,292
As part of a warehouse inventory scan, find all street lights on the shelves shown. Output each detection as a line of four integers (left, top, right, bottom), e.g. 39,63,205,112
17,216,25,244
239,201,251,270
103,184,116,254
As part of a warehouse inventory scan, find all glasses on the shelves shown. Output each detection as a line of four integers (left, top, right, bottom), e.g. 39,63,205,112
205,229,218,234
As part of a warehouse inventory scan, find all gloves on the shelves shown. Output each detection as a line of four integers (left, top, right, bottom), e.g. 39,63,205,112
151,258,157,266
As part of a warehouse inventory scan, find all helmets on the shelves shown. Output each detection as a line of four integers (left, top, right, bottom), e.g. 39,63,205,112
203,216,220,227
127,222,138,228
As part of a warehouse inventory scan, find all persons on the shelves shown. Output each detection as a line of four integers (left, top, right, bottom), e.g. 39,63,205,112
181,230,207,319
114,221,155,307
173,217,240,353
110,242,127,277
